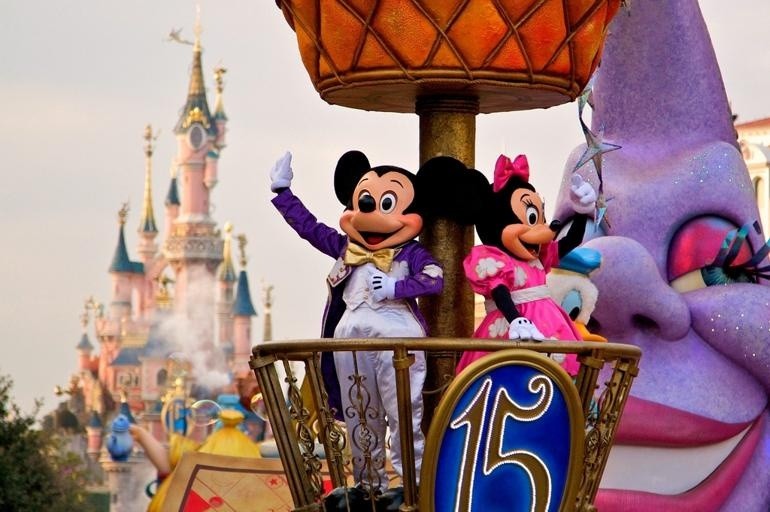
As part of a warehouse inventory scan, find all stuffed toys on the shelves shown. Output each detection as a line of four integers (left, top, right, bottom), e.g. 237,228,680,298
446,151,599,380
266,148,469,511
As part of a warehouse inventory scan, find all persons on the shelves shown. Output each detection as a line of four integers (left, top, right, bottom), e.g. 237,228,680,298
544,0,770,509
